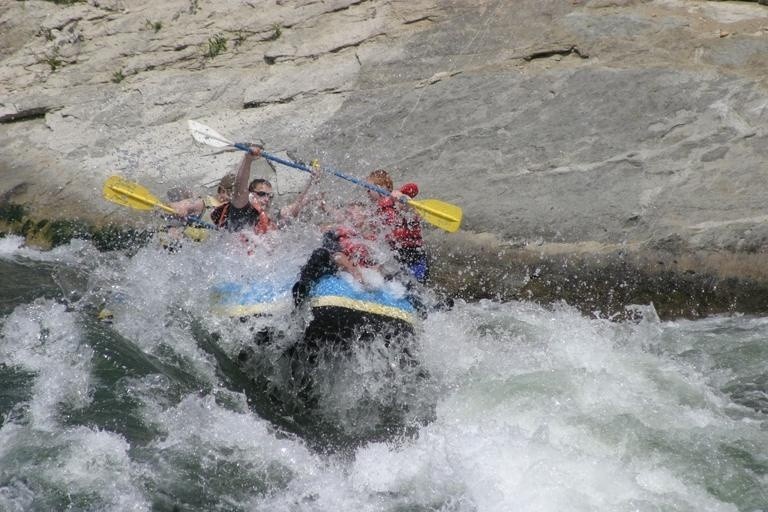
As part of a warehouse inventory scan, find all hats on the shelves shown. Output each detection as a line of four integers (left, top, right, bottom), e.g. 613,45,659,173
221,173,234,190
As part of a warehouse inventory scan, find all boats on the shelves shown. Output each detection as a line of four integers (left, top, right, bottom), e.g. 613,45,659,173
176,217,417,354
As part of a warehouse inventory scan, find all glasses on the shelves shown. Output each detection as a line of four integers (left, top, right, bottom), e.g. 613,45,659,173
251,190,274,199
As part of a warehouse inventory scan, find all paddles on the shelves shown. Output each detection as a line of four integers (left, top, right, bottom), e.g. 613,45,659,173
188,120,463,233
104,176,219,231
